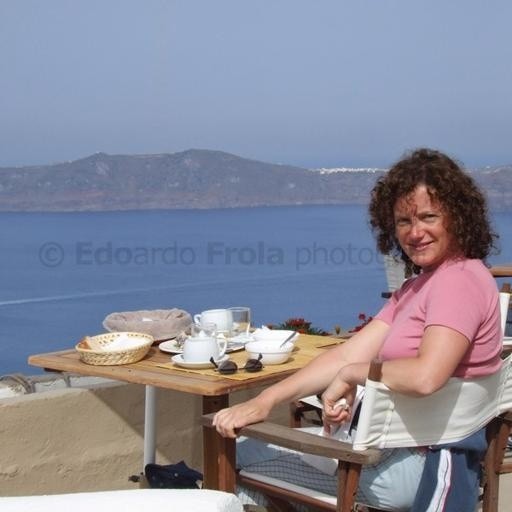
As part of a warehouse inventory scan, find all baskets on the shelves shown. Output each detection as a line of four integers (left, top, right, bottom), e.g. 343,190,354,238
76,330,155,365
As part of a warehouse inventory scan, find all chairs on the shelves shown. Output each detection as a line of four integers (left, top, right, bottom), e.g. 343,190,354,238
199,347,512,512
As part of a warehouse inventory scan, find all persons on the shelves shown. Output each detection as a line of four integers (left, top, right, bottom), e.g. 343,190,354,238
211,149,503,512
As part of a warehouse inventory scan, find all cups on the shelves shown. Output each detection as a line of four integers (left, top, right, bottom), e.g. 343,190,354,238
193,308,234,332
181,335,228,361
186,322,217,337
226,306,251,340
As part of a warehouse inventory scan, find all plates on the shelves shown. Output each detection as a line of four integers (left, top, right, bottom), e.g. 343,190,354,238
172,355,232,369
157,337,249,354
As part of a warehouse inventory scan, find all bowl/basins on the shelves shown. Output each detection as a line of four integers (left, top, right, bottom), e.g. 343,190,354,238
245,341,295,365
254,328,299,345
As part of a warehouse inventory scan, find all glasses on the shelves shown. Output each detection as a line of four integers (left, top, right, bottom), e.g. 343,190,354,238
210,353,262,373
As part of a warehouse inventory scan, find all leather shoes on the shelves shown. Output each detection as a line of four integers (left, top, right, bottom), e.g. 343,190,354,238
145,460,203,489
